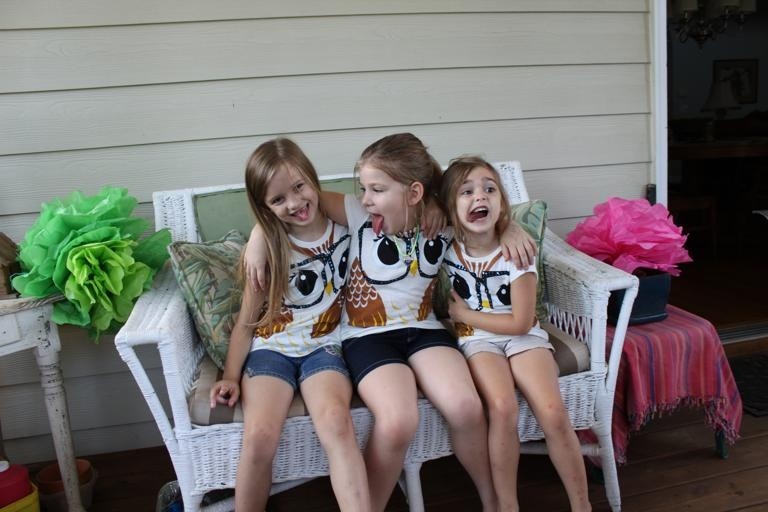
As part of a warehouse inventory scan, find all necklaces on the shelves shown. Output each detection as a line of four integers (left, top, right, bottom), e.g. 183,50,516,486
394,225,419,260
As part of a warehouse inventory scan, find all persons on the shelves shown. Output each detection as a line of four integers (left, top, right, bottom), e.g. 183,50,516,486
443,155,590,511
211,136,446,511
243,131,539,511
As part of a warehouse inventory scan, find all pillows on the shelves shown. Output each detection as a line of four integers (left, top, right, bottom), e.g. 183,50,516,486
192,178,362,245
438,199,548,320
167,228,245,372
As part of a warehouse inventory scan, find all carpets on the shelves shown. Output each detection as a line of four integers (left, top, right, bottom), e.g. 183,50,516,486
727,352,768,417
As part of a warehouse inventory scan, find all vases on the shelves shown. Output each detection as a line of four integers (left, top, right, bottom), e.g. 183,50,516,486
608,273,670,324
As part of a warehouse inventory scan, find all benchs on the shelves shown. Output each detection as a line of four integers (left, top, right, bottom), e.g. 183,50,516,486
115,160,639,512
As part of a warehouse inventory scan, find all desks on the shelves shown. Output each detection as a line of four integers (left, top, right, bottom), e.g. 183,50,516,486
0,291,86,512
541,304,742,480
